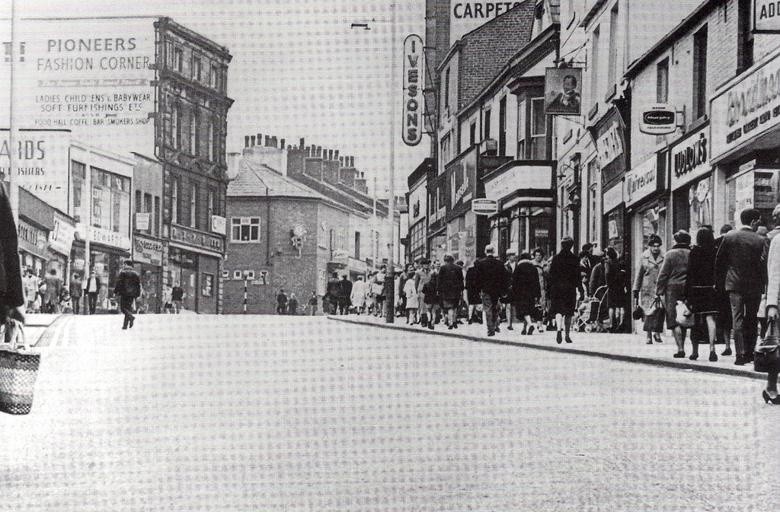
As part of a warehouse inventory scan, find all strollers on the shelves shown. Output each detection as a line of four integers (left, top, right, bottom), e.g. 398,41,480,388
573,284,610,332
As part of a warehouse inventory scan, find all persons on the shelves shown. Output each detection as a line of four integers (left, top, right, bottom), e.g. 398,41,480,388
634,202,779,406
289,294,299,315
327,265,386,318
309,291,318,316
546,75,580,113
20,267,184,315
277,288,287,314
395,236,626,343
114,259,141,330
1,179,27,340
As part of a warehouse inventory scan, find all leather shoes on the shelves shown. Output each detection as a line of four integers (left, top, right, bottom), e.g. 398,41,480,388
674,348,753,364
646,335,662,344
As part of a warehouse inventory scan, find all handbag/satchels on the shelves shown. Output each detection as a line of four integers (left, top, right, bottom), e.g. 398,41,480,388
676,301,695,327
753,345,779,372
633,307,645,320
0,318,40,415
652,308,666,328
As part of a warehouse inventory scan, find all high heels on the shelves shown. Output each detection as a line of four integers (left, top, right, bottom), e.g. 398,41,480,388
763,389,779,405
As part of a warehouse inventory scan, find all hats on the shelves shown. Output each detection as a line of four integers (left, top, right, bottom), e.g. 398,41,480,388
674,230,690,244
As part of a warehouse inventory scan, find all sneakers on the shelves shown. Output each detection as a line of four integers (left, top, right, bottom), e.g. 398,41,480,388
488,324,500,336
557,330,573,343
521,319,553,335
422,319,473,330
